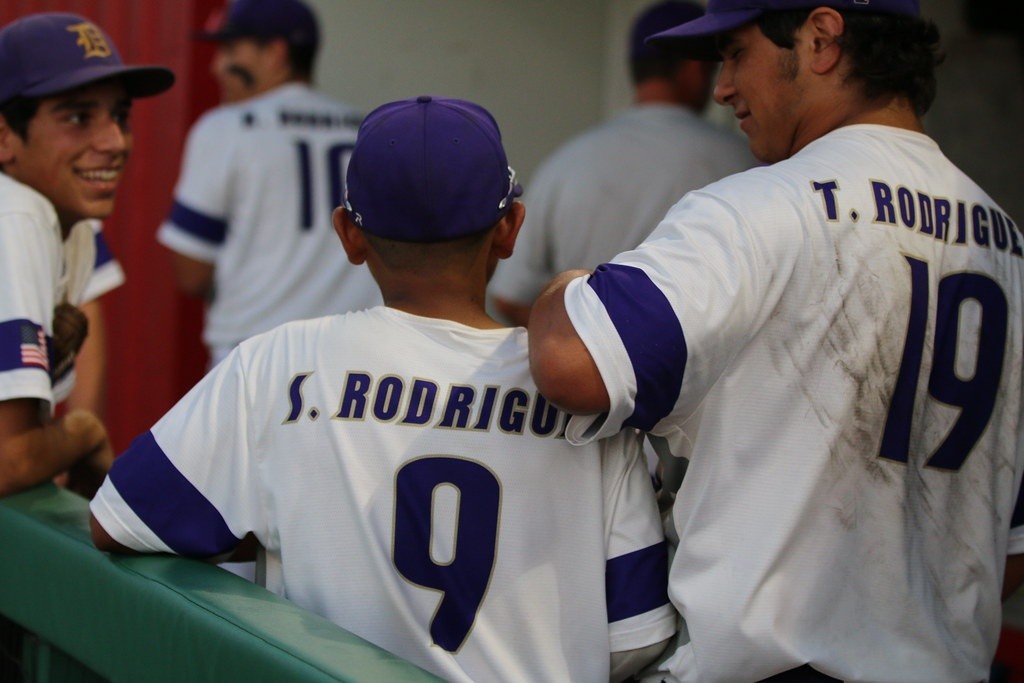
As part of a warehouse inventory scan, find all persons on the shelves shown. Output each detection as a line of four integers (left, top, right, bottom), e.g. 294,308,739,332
156,1,388,371
87,95,681,683
1,13,179,498
530,1,1023,682
485,2,765,331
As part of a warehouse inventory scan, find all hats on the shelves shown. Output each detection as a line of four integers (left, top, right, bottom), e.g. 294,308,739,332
0,13,176,101
645,0,922,62
344,96,524,237
192,0,317,45
